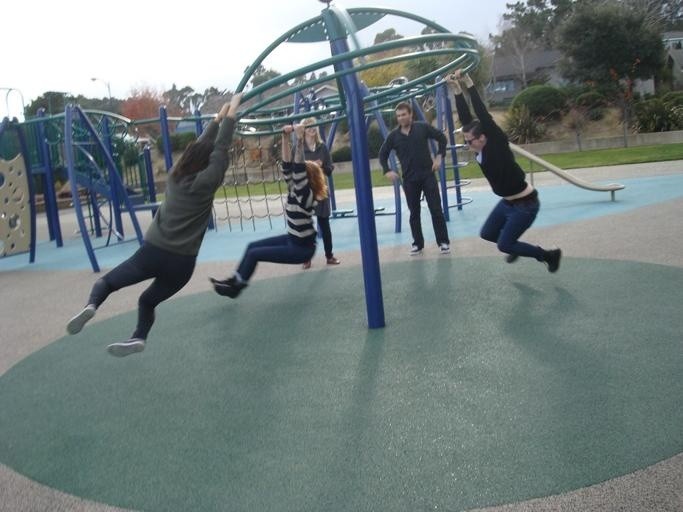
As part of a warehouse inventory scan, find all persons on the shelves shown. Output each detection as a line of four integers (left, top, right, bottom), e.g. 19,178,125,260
291,116,340,270
64,90,245,358
209,120,327,299
446,68,561,271
378,102,450,255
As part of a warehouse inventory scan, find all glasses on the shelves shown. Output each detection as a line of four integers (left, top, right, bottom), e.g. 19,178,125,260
464,137,477,145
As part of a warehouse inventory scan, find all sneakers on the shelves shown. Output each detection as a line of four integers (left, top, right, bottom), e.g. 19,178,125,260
66,303,96,336
210,274,247,299
303,256,340,269
105,337,146,358
505,248,561,273
408,243,450,254
